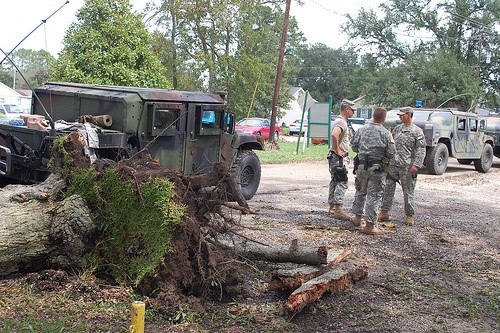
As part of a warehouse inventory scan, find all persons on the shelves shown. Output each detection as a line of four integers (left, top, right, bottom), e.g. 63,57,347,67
326,99,357,221
350,107,396,235
376,106,426,225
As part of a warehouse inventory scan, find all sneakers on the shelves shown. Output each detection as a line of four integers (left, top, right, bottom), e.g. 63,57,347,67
407,216,414,226
378,210,389,220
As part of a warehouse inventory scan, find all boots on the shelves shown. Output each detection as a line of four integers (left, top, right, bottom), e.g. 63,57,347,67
329,204,352,220
350,214,361,226
364,222,383,234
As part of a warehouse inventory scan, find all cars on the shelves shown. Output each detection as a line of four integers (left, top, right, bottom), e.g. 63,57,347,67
289,119,308,136
235,117,280,139
0,102,25,118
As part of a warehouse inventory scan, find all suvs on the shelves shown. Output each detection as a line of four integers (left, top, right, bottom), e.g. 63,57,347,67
364,109,495,175
0,82,264,200
457,113,499,164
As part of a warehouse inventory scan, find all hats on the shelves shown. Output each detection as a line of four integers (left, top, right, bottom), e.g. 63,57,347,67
341,99,358,111
397,108,414,115
